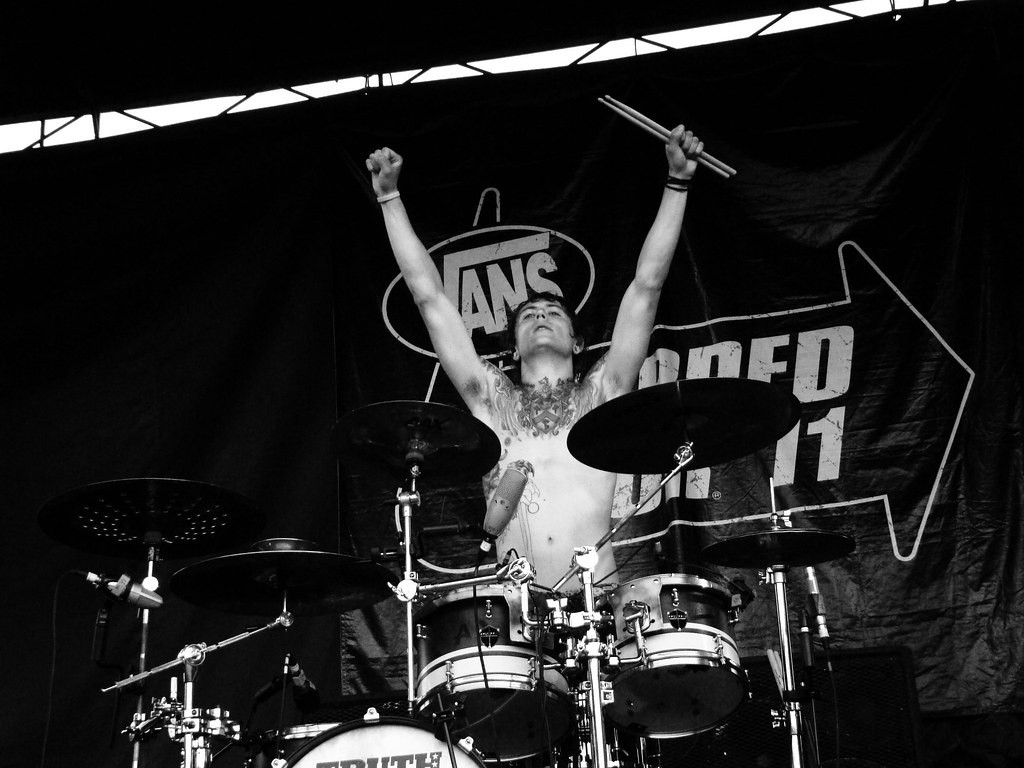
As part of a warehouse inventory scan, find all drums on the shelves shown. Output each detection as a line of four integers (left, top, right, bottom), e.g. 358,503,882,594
591,570,752,742
204,714,489,768
409,582,576,765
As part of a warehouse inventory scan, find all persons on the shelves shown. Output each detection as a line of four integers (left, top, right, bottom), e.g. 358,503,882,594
365,125,704,643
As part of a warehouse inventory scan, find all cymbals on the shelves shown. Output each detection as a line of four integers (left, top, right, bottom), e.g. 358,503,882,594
565,374,805,477
36,475,270,564
698,522,858,570
325,398,503,487
168,537,402,624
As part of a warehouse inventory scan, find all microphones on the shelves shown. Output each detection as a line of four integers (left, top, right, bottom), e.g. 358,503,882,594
476,468,528,568
805,566,829,653
502,551,511,568
77,571,163,609
288,654,316,692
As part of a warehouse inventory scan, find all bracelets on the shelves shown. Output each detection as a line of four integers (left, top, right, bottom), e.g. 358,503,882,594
666,175,689,191
377,192,400,203
